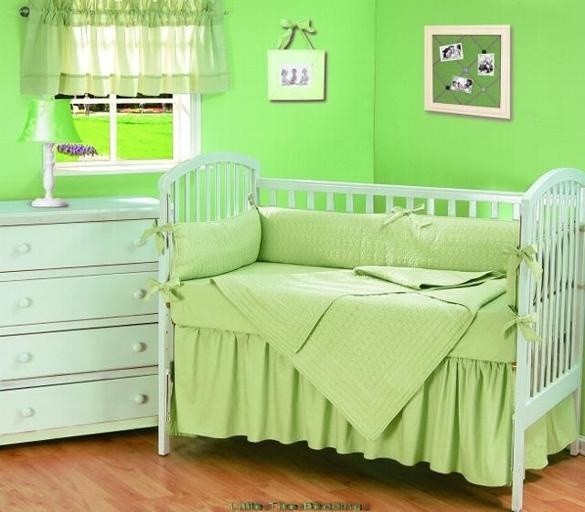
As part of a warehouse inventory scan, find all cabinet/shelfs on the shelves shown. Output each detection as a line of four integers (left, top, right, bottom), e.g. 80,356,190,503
0,195,167,452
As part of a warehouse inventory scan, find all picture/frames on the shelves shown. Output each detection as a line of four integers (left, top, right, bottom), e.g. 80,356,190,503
266,46,326,104
420,24,515,123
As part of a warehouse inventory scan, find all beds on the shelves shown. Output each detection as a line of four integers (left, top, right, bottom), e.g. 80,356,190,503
154,148,585,511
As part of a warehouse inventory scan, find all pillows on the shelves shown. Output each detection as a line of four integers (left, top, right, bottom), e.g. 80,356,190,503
166,206,263,280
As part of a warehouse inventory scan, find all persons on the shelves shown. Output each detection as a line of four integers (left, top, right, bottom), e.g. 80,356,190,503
443,46,460,58
283,68,306,85
453,80,471,91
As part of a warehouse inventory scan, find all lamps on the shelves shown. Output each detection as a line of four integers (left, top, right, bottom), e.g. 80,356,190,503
15,96,83,206
